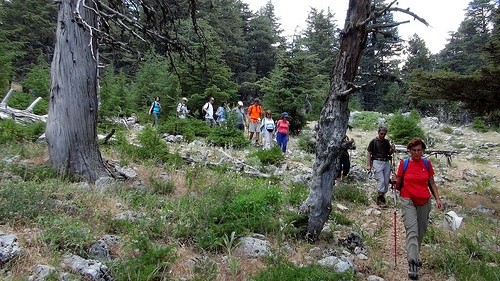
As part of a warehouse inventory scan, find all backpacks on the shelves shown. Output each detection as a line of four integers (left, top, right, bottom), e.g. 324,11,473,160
199,102,209,119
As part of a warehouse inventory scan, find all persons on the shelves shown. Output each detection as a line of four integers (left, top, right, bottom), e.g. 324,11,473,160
202,96,216,126
176,97,190,119
366,127,396,209
260,110,275,150
275,112,290,154
215,101,230,129
248,98,262,147
230,101,247,132
336,137,355,186
149,96,162,124
391,139,445,280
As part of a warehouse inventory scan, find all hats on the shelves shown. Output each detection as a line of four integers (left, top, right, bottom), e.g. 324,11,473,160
282,112,288,117
182,97,187,102
238,101,244,107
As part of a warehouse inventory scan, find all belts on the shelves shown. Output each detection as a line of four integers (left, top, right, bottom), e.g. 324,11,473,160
372,158,389,161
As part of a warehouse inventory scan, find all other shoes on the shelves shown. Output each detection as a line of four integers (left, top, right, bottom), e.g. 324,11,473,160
377,195,387,208
407,259,422,280
342,176,348,183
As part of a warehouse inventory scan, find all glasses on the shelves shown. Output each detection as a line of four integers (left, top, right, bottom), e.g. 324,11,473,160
379,131,387,136
